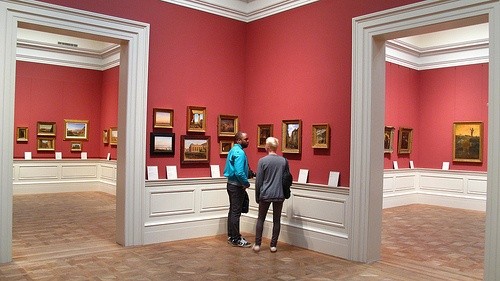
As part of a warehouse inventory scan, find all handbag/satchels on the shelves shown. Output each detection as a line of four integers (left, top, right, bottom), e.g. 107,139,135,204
242,192,249,213
282,157,292,199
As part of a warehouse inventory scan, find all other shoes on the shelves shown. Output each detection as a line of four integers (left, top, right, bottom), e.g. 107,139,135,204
231,238,251,247
227,236,245,243
253,243,261,252
270,247,277,253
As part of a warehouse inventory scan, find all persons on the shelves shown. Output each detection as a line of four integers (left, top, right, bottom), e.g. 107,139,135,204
223,131,254,247
252,137,289,253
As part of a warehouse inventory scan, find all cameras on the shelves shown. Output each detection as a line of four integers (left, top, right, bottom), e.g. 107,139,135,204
249,170,256,178
